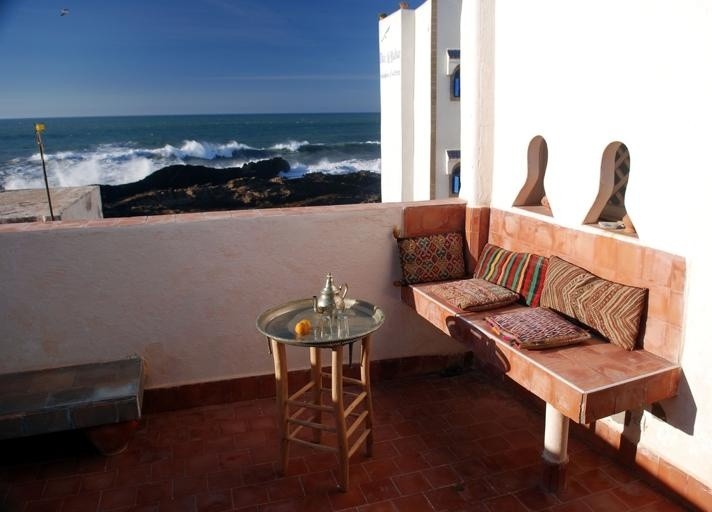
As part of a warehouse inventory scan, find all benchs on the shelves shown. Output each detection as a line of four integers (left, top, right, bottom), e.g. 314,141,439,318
397,200,688,495
0,355,149,456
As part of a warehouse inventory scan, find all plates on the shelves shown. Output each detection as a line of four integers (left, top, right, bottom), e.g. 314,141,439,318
599,221,622,229
255,296,385,347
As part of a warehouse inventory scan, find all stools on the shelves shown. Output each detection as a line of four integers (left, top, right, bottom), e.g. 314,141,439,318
270,332,374,493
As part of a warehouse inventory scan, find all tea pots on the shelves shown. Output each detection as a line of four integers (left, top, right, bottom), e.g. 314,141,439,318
309,273,348,317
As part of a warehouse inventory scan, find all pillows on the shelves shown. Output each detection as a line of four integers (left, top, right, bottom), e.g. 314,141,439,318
427,278,520,313
539,254,648,350
392,225,467,285
483,306,592,350
472,242,548,309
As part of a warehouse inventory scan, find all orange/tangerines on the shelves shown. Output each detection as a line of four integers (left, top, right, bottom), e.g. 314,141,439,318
296,320,311,334
